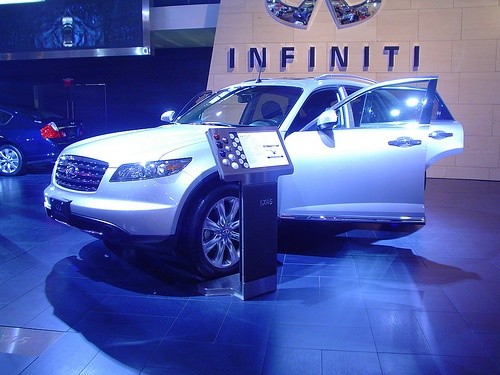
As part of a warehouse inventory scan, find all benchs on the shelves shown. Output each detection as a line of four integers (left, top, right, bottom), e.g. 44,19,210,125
295,101,379,130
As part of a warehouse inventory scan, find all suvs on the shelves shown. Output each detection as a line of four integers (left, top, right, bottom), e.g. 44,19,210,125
43,73,465,282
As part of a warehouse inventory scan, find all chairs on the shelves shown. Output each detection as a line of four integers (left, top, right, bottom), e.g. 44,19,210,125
261,101,284,126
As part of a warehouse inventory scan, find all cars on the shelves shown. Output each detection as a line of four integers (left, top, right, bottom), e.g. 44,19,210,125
0,104,86,177
